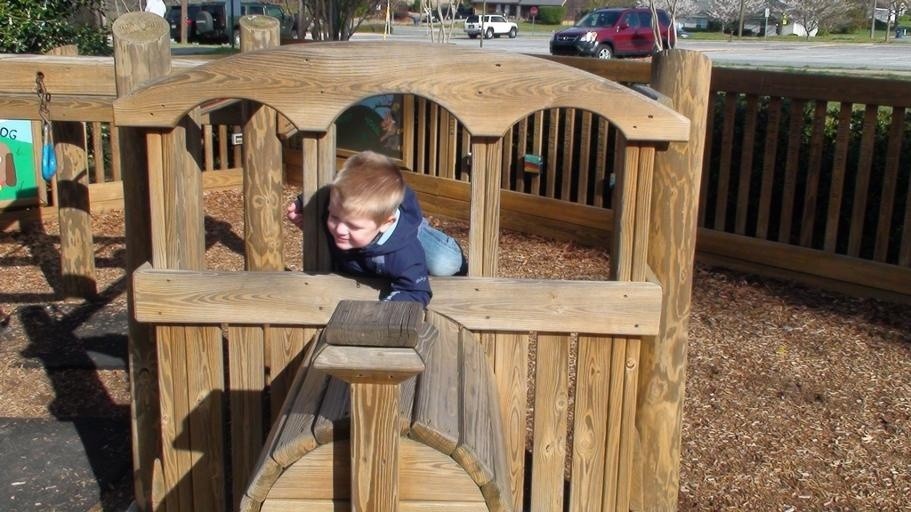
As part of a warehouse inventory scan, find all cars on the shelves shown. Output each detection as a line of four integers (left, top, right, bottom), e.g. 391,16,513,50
676,29,689,39
422,7,462,23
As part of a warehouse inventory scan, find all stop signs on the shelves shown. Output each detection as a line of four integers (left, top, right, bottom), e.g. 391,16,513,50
529,6,538,16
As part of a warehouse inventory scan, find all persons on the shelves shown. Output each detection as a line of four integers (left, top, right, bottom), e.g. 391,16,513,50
288,151,467,310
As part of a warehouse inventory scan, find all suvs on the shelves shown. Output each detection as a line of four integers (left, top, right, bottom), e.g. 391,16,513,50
164,1,296,46
549,8,675,59
463,14,518,40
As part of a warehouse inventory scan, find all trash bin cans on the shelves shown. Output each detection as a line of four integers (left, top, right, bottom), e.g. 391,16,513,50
895,27,903,38
